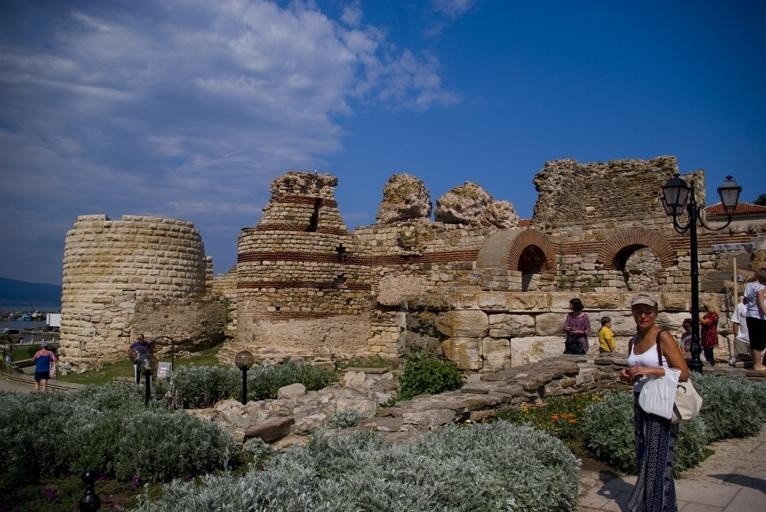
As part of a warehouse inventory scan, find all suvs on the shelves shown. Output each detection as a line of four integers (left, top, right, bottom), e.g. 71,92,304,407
0,328,22,343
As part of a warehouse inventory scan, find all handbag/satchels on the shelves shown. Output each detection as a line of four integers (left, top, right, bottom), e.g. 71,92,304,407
671,379,703,422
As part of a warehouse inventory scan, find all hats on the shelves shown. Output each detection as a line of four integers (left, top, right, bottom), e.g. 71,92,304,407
632,292,658,307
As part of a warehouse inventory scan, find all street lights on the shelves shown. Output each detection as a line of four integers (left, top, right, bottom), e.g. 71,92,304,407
659,173,742,377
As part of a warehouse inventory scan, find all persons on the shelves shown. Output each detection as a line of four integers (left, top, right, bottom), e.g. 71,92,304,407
699,300,719,366
32,342,57,392
730,299,752,361
598,317,615,352
743,267,766,372
563,298,591,354
619,292,689,512
128,334,153,385
